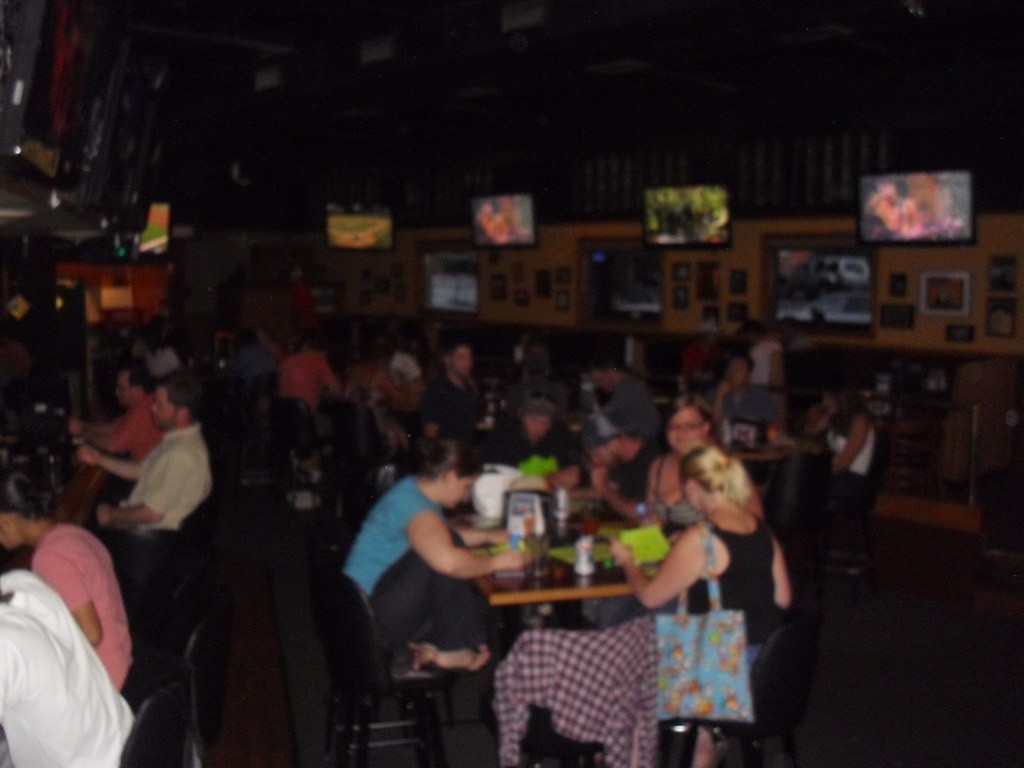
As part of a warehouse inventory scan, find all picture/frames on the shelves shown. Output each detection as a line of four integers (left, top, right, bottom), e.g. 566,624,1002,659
919,270,970,317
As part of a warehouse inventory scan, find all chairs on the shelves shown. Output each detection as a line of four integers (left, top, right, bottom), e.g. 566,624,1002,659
122,494,237,768
272,391,889,768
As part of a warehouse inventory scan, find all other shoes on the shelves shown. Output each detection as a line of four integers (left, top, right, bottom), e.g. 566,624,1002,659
285,488,322,511
411,642,494,676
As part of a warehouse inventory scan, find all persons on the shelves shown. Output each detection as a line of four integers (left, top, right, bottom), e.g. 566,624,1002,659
0,268,877,768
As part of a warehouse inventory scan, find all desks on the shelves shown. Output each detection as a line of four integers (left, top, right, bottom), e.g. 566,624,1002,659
452,517,653,606
0,440,112,570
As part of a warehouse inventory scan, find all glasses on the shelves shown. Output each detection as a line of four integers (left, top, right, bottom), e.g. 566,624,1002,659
666,420,705,432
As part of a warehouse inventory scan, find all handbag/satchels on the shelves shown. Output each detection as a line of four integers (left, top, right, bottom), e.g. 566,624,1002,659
654,521,755,723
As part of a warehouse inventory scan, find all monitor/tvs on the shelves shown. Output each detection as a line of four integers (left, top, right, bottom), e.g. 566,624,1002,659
851,166,980,249
470,191,540,250
641,182,733,252
117,203,173,263
324,202,395,252
